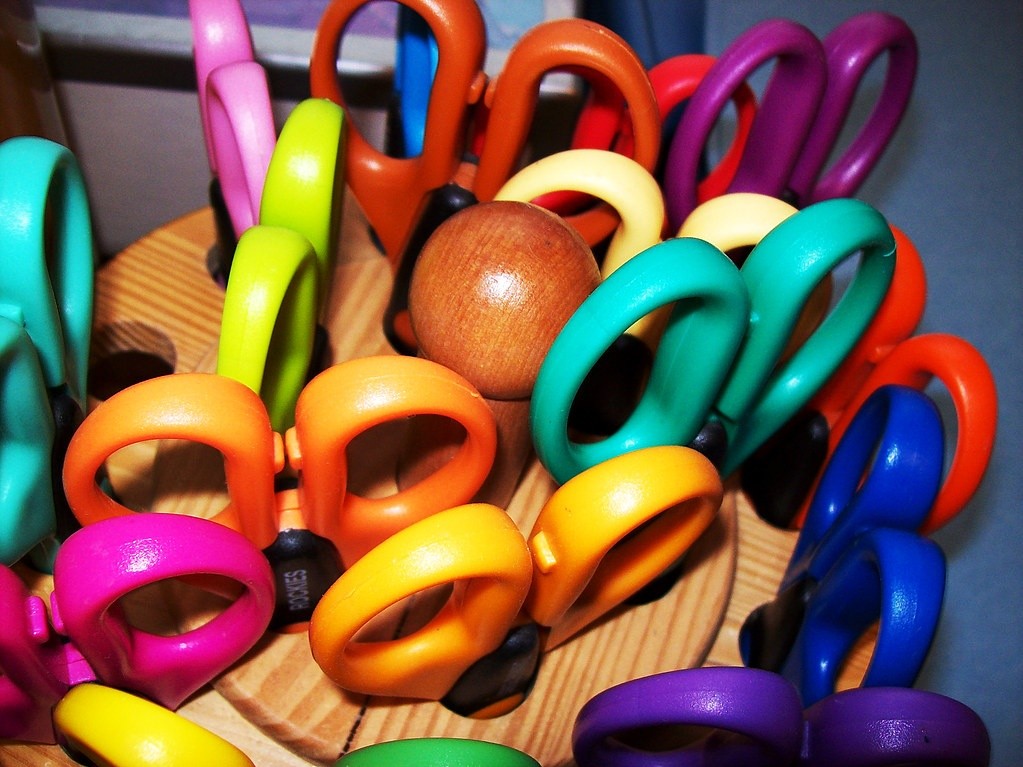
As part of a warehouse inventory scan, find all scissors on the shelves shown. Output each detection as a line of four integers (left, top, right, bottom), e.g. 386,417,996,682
0,0,1000,766
